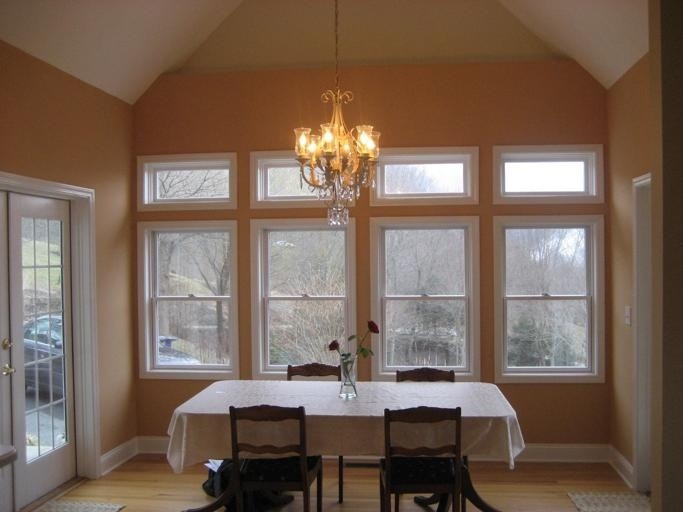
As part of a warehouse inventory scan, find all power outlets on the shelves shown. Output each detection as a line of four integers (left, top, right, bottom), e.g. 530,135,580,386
623,305,633,327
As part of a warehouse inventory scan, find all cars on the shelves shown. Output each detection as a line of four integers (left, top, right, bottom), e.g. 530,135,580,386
21,310,206,406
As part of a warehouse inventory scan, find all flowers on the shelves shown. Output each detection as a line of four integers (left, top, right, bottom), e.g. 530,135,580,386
327,320,381,393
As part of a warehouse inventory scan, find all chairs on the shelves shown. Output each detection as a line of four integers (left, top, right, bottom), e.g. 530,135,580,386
397,366,454,382
287,363,344,502
379,405,466,512
229,400,323,511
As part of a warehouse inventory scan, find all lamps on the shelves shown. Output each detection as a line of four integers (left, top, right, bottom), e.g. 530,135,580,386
293,1,382,227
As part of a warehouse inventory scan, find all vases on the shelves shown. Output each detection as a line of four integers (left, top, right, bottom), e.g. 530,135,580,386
338,352,358,399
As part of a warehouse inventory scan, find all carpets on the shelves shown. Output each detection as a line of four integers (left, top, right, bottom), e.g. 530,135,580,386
565,488,654,511
